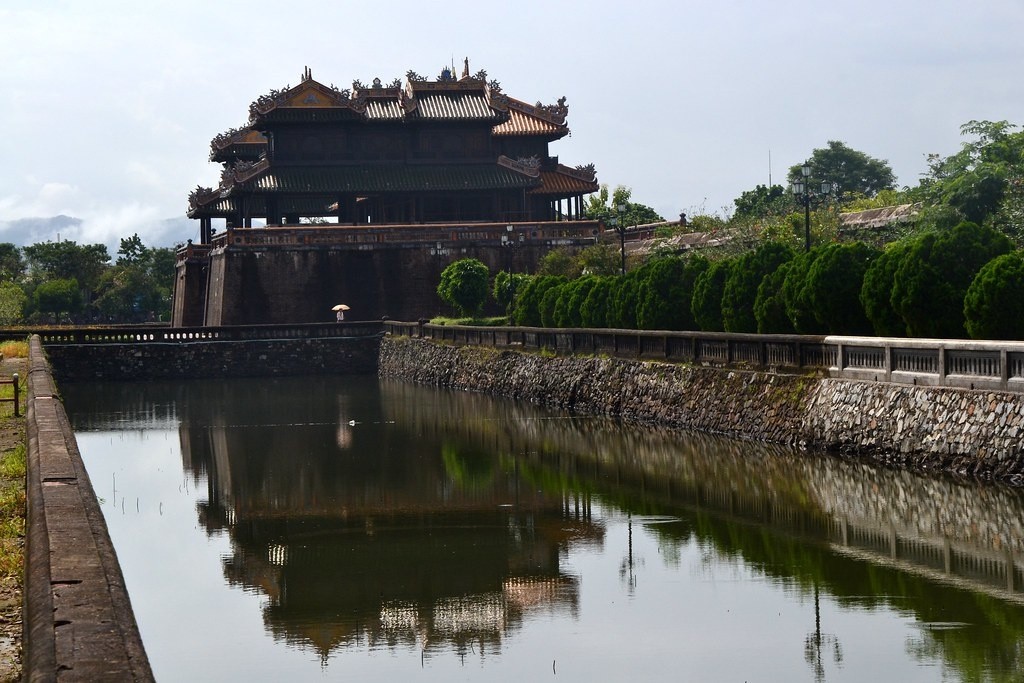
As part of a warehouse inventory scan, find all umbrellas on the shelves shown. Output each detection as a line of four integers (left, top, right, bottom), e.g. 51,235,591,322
332,305,350,310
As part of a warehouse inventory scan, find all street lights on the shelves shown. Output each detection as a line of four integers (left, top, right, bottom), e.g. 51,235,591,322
500,221,524,328
610,201,638,279
619,492,647,604
804,517,844,682
790,161,829,250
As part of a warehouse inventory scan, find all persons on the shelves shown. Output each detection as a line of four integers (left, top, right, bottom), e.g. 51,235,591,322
337,308,344,323
